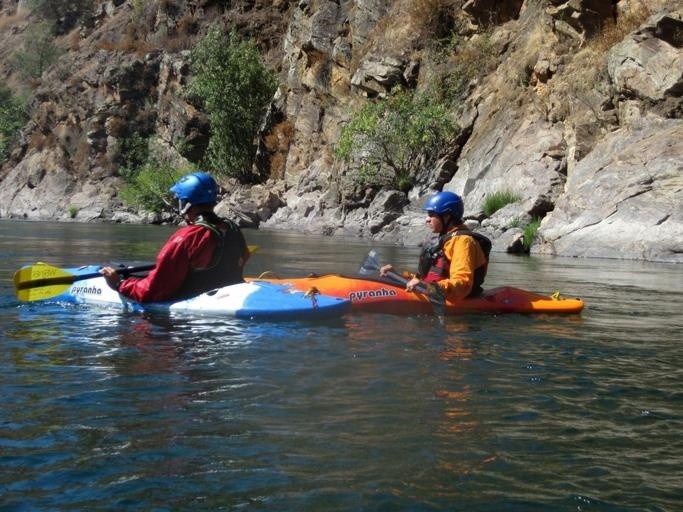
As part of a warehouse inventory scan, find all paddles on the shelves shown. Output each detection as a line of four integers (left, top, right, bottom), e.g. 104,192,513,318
359,248,445,318
13,245,260,302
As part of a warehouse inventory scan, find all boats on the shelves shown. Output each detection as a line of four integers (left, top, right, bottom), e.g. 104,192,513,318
241,272,585,315
18,262,352,322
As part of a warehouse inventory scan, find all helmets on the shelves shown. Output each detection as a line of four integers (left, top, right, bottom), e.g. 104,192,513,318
170,172,218,205
422,191,465,220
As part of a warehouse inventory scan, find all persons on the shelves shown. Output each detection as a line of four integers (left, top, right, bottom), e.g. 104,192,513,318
99,173,250,304
380,192,492,299
